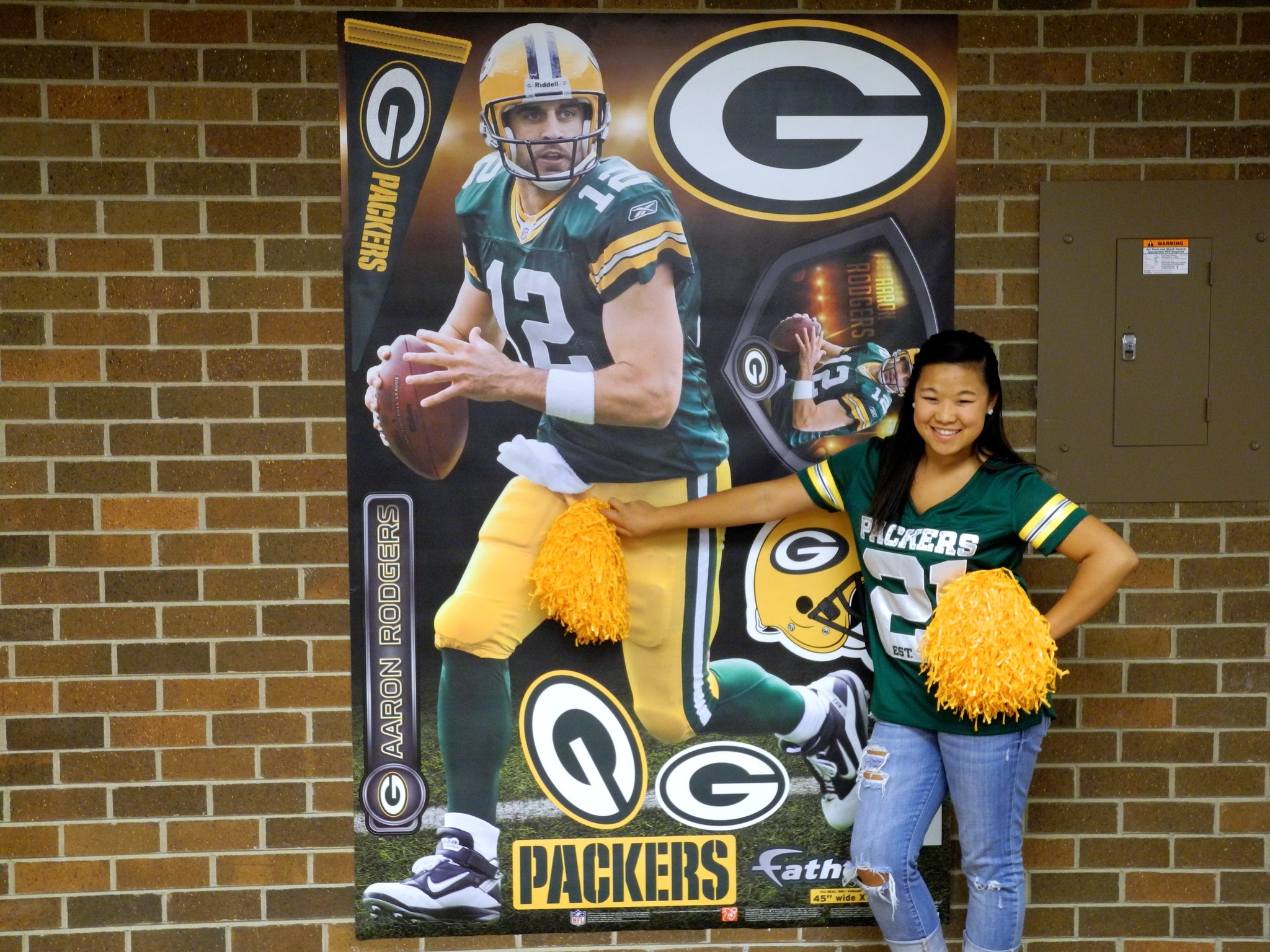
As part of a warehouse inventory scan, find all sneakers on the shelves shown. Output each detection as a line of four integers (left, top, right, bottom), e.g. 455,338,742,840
363,828,504,928
777,669,869,831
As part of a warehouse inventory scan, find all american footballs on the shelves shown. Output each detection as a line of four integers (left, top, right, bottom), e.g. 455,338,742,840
769,317,822,352
378,335,470,482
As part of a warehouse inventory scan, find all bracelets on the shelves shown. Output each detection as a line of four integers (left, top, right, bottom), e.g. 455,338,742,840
545,369,595,425
792,380,813,400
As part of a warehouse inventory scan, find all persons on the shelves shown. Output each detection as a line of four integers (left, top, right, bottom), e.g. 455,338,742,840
362,22,878,923
776,313,921,449
599,329,1139,952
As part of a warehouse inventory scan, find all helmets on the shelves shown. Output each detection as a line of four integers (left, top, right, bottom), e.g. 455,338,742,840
878,347,920,397
479,22,610,191
